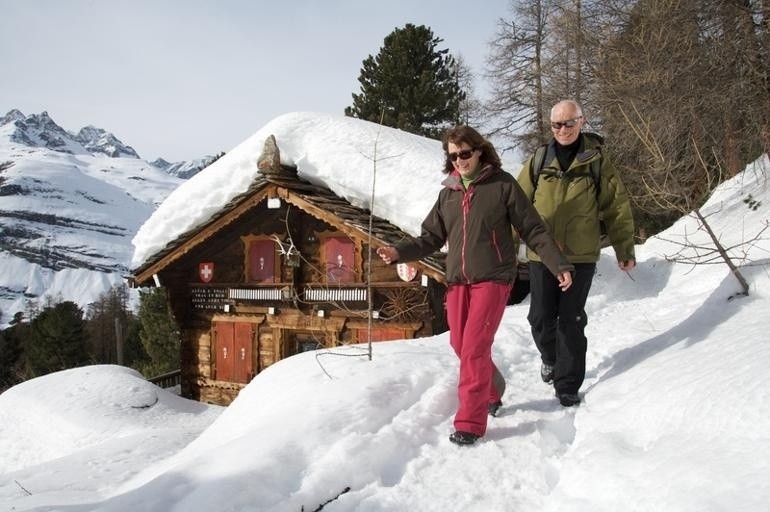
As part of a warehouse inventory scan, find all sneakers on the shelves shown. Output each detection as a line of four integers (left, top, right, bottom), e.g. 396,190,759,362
449,432,478,445
559,389,581,408
484,399,505,414
540,361,558,384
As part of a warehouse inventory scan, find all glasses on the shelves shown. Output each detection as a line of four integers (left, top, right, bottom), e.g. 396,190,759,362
550,115,583,128
448,149,481,159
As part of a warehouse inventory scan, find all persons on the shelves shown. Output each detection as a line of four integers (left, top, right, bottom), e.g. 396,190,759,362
378,124,576,443
510,98,638,407
